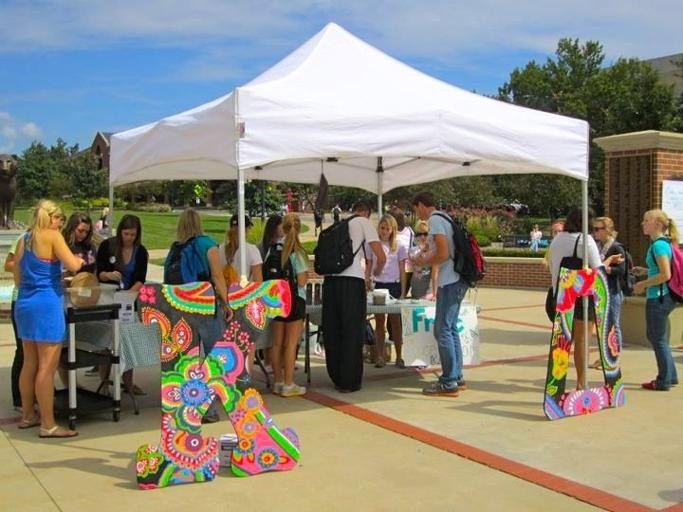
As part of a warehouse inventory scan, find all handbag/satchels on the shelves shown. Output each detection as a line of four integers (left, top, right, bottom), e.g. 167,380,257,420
550,233,583,316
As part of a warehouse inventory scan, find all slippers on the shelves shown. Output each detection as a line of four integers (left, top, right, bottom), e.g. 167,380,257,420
122,385,147,395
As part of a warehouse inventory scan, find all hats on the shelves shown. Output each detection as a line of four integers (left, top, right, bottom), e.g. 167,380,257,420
230,213,254,227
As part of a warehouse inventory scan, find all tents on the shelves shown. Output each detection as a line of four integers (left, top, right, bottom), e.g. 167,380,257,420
107,20,591,390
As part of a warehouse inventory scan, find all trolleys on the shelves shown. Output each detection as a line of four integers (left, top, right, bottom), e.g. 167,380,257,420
52,301,123,430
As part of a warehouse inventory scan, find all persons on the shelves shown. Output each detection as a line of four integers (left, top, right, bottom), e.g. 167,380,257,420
61,204,98,278
219,214,264,377
96,206,149,396
263,212,309,397
386,205,414,341
314,207,323,236
587,216,626,371
13,198,87,438
546,205,622,391
529,223,543,251
163,207,234,423
97,207,110,230
551,216,566,238
411,191,470,397
631,208,679,391
331,203,342,223
408,220,432,299
320,199,388,393
365,208,407,369
256,214,286,374
4,213,67,414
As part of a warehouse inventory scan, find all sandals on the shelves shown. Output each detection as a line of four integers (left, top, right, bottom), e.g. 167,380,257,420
38,424,79,438
18,414,41,429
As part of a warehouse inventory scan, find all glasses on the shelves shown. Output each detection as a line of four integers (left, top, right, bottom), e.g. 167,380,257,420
416,232,428,237
593,226,605,232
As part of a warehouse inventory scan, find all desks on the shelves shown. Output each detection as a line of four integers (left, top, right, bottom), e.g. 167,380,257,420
303,297,437,383
1,284,122,432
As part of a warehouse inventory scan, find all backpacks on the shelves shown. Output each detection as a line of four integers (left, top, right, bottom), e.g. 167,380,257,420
262,243,298,313
650,236,683,304
313,214,366,276
432,212,488,289
333,207,340,221
611,241,636,298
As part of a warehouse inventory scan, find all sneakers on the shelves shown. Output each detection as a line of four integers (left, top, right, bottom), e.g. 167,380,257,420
84,365,100,376
272,382,286,394
589,359,602,368
641,379,659,388
280,382,307,397
375,359,386,368
395,358,406,368
457,379,467,391
422,384,460,397
